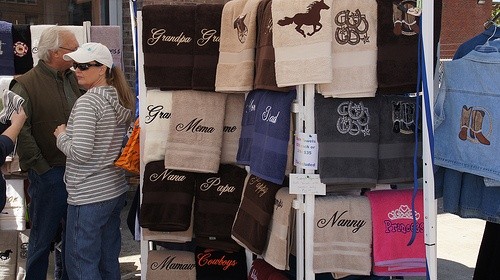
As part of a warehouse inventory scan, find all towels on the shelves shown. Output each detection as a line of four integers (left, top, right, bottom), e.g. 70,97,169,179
1,2,427,280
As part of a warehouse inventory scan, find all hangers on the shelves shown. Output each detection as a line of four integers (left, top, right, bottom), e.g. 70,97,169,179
475,20,499,54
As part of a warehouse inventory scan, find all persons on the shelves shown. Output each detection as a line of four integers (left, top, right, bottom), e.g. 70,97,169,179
53,42,136,280
0,106,28,214
8,26,88,280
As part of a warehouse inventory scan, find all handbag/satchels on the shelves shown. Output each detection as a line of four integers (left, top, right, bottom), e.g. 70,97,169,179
114,117,140,175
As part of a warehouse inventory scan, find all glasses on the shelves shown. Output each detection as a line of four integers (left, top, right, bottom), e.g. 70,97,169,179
59,47,77,52
72,63,101,71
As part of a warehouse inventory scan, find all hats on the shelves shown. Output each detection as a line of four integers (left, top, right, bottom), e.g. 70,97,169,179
62,42,113,73
